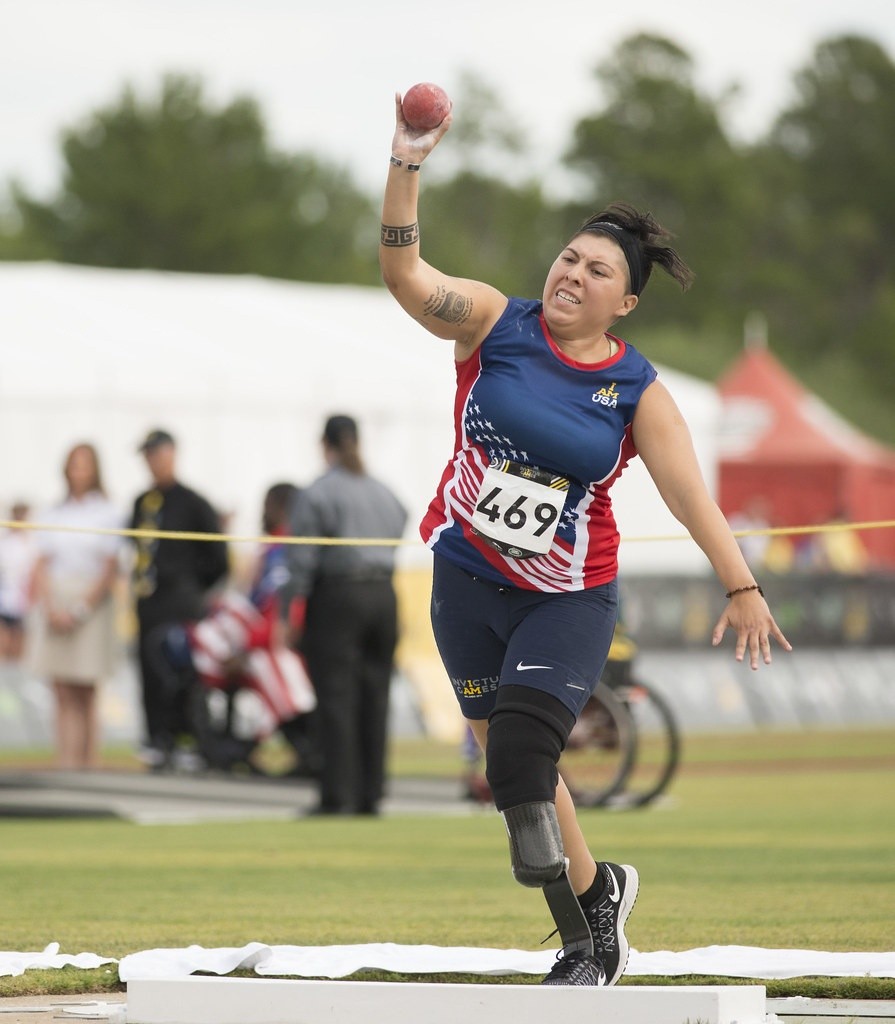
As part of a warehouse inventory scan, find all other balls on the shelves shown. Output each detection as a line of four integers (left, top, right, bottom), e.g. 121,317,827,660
402,81,450,130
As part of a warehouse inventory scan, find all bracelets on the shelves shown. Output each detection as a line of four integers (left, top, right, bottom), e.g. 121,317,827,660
725,586,764,602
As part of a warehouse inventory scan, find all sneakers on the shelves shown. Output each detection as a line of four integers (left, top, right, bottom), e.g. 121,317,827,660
540,945,608,986
540,861,640,987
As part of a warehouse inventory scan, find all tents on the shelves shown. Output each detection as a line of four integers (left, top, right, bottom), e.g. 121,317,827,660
694,307,895,573
1,259,740,645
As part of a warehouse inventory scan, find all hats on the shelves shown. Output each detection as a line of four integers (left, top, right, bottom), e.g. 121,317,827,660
137,431,170,451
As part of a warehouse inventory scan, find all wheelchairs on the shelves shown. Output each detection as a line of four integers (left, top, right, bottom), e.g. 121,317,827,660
550,655,683,811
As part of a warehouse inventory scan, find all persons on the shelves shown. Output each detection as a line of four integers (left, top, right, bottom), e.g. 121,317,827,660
377,83,794,988
1,418,409,816
